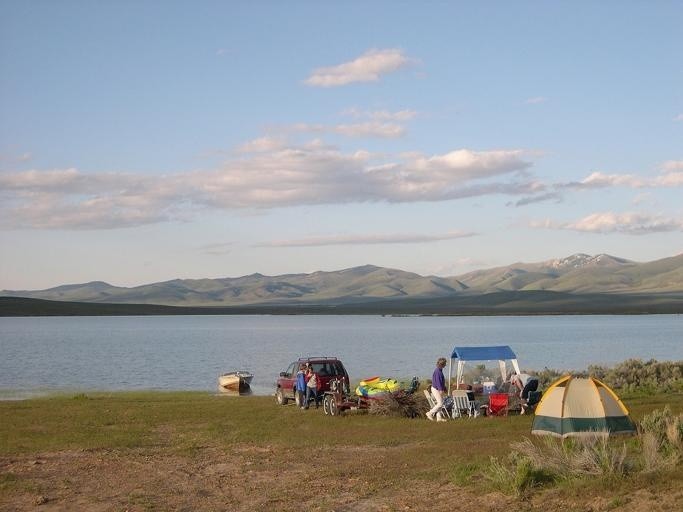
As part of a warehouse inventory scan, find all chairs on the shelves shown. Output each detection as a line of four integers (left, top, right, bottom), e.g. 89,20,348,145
422,388,542,421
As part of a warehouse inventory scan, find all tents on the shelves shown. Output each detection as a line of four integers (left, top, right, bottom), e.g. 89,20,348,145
529,376,638,438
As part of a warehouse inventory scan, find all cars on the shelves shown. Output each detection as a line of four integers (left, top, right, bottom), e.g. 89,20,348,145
276,356,349,405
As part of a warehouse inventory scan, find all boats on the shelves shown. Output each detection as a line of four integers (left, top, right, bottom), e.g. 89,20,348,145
219,371,254,392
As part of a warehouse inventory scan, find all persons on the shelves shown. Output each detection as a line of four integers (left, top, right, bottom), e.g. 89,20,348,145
303,367,321,410
476,365,489,384
506,372,538,415
463,385,475,415
426,358,448,422
409,376,421,392
295,364,306,408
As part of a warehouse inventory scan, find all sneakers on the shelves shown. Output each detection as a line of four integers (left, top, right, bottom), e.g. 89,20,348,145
298,405,320,409
425,412,434,421
436,417,448,422
